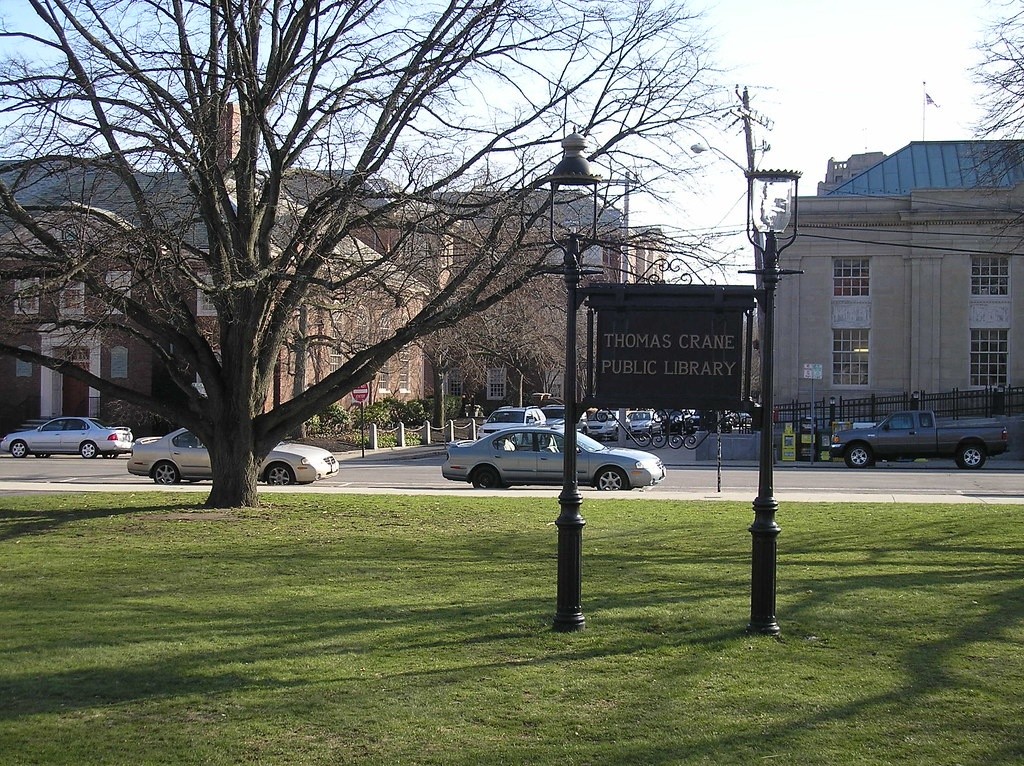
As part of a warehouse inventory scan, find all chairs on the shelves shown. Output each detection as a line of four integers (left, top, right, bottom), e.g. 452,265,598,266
541,438,555,454
496,439,511,450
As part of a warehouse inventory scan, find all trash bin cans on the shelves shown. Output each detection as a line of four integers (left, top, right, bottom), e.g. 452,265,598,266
799,417,818,461
832,421,851,462
781,422,796,461
816,429,832,462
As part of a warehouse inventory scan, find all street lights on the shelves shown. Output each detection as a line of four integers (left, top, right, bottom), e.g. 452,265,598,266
689,141,752,176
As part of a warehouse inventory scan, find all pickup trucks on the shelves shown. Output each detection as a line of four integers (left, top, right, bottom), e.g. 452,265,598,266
829,410,1010,469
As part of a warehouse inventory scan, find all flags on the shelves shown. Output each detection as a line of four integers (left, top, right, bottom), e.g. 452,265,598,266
925,92,940,107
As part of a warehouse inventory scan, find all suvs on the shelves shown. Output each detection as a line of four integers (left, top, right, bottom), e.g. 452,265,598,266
477,405,546,445
539,405,588,433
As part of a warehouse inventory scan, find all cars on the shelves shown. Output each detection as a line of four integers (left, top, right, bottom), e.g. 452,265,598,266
660,402,761,436
626,411,663,436
586,409,632,441
1,416,137,460
127,427,340,487
442,426,668,493
